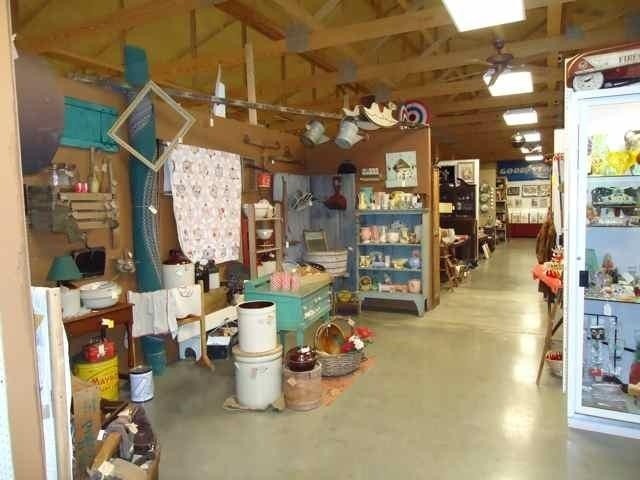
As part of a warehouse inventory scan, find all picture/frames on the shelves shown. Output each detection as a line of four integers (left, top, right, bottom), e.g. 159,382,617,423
457,162,475,184
507,184,551,224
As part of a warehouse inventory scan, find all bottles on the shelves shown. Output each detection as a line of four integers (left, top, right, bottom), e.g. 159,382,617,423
195,259,219,293
406,224,416,243
357,192,367,211
371,276,379,291
47,163,58,186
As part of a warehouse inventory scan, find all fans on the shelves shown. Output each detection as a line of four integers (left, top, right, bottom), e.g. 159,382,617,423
465,40,563,87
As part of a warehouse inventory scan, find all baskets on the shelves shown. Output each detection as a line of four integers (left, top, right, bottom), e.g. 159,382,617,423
312,315,365,377
545,351,563,377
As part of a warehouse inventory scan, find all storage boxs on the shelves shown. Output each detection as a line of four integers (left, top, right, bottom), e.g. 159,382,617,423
206,326,240,360
72,377,102,480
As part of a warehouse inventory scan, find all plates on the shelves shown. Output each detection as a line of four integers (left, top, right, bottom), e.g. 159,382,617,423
360,276,373,290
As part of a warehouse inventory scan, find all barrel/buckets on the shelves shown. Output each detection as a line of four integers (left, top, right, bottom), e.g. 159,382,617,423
129,367,154,403
232,345,284,407
71,355,120,401
237,300,277,354
163,264,196,291
285,363,323,411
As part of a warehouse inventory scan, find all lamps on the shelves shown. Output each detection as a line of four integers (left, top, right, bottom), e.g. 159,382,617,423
299,114,359,150
46,255,81,290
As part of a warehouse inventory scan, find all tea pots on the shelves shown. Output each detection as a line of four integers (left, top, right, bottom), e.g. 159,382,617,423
334,115,370,151
300,118,331,148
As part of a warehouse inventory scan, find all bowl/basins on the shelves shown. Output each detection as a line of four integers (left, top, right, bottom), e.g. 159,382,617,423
385,233,400,243
392,258,407,269
255,229,274,240
80,280,121,310
242,203,267,217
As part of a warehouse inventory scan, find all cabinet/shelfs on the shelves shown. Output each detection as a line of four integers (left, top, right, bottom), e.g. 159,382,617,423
478,225,495,258
496,176,508,241
240,201,283,279
563,83,640,441
354,207,431,317
439,177,478,266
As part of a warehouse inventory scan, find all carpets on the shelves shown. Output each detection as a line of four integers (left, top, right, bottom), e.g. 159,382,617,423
282,353,377,411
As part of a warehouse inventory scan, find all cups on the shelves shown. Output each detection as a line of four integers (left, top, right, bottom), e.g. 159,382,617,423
359,256,370,267
270,271,300,290
263,206,275,218
409,279,420,294
373,191,392,210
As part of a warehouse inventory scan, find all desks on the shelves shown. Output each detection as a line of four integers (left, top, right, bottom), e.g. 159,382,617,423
529,264,564,386
440,235,471,289
243,272,334,359
59,301,135,381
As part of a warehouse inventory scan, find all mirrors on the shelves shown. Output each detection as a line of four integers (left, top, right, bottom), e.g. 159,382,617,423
241,156,258,193
